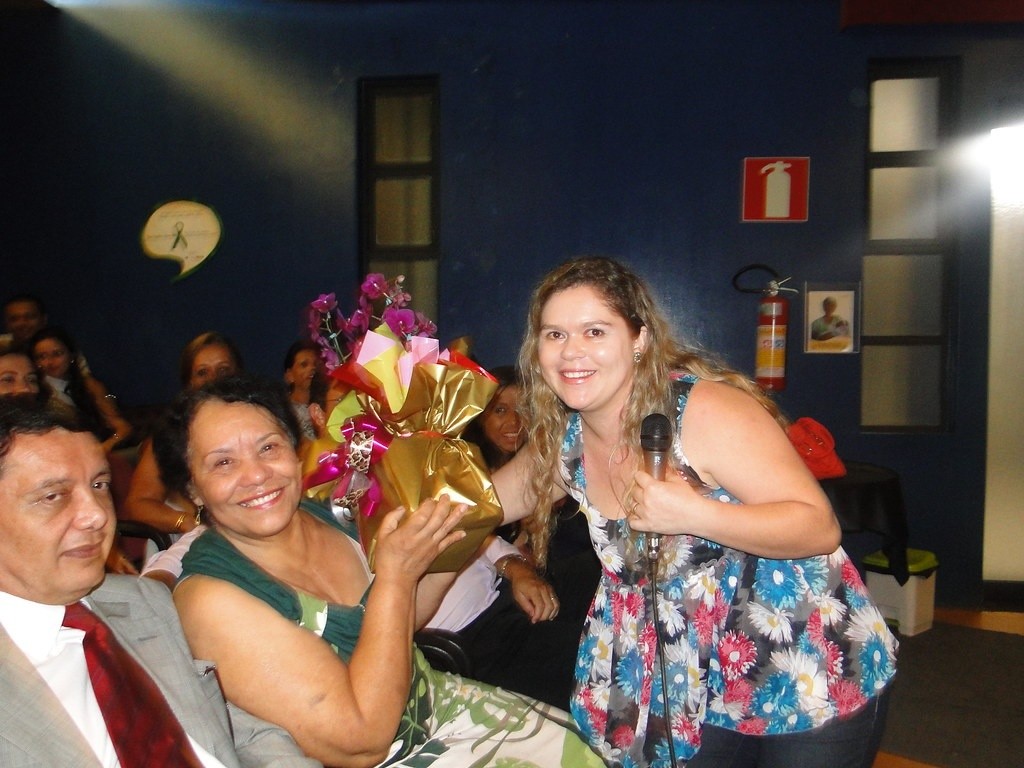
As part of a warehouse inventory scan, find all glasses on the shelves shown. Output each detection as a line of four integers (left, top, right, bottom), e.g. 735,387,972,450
326,397,346,403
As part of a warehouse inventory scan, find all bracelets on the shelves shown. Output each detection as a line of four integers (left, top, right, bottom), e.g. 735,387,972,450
498,554,528,577
172,512,188,533
113,433,121,442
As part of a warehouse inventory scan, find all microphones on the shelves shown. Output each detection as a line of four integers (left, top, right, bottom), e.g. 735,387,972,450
641,413,673,575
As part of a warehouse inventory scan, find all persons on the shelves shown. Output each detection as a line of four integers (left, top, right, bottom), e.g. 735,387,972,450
0,394,323,768
171,375,608,768
0,287,603,768
480,257,898,768
810,294,849,340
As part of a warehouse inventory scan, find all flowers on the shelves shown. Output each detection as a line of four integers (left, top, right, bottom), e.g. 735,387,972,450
311,273,500,575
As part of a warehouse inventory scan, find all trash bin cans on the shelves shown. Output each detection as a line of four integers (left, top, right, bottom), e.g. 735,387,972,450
862,547,938,637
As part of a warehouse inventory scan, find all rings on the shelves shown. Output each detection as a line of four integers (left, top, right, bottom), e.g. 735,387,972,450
633,503,639,514
551,596,557,600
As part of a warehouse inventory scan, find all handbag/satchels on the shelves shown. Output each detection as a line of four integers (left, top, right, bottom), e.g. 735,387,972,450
788,417,847,479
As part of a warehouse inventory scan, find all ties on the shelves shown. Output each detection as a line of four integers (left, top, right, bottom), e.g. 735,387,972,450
62,601,205,768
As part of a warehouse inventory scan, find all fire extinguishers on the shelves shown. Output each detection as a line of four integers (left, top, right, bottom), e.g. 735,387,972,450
733,264,799,391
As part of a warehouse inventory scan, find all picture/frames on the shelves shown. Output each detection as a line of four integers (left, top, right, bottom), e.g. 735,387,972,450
803,281,860,355
